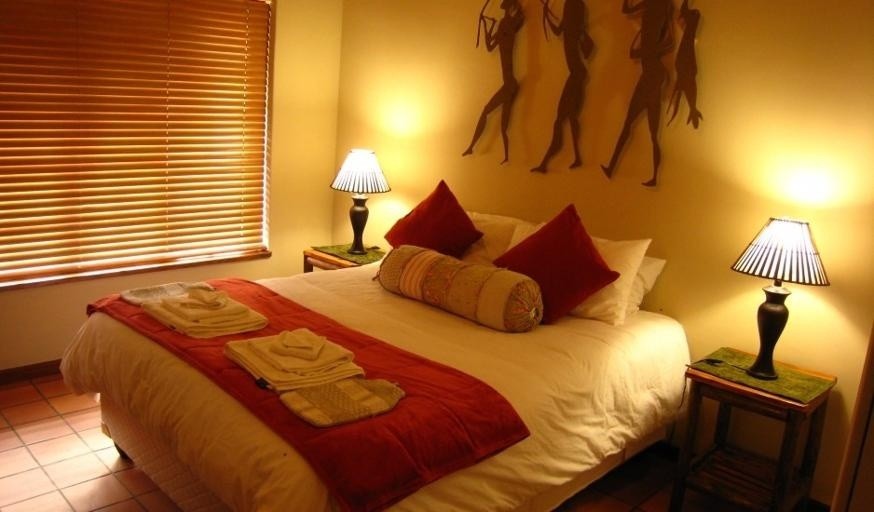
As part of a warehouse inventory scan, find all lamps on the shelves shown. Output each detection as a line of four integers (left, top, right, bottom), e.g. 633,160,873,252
329,144,394,256
727,209,836,380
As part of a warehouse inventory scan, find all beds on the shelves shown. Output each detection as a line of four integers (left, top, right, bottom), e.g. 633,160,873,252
58,211,701,509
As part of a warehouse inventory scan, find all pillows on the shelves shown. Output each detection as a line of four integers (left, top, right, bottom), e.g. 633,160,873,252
368,244,545,334
382,174,486,263
457,203,665,325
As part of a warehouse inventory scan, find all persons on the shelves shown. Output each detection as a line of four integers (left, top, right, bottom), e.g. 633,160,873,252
462,0,525,165
598,0,676,187
530,1,593,175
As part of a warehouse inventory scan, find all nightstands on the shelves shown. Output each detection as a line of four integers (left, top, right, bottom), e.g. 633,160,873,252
668,344,838,511
298,239,387,276
